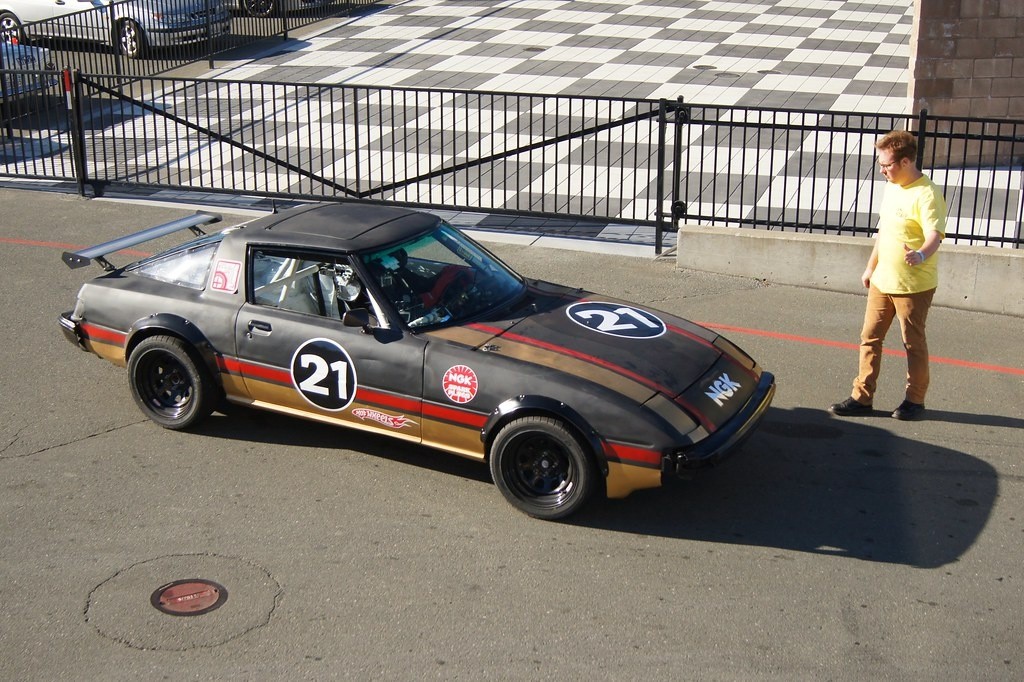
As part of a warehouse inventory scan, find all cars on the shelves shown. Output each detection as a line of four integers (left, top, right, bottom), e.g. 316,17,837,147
0,0,232,60
221,0,335,18
0,34,58,104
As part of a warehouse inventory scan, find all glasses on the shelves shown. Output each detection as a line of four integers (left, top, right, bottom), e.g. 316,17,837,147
875,155,895,168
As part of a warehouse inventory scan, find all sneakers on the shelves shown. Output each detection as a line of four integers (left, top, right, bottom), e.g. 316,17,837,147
830,396,872,417
891,400,926,419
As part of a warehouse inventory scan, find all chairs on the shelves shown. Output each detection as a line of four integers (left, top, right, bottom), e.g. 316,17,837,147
374,248,422,316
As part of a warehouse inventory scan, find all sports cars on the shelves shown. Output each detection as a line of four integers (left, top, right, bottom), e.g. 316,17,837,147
56,202,776,526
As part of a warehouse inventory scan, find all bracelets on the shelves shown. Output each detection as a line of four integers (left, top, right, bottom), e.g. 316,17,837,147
915,250,925,262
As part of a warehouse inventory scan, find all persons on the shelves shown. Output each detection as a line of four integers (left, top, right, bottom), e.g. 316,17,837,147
368,249,475,328
831,129,947,420
